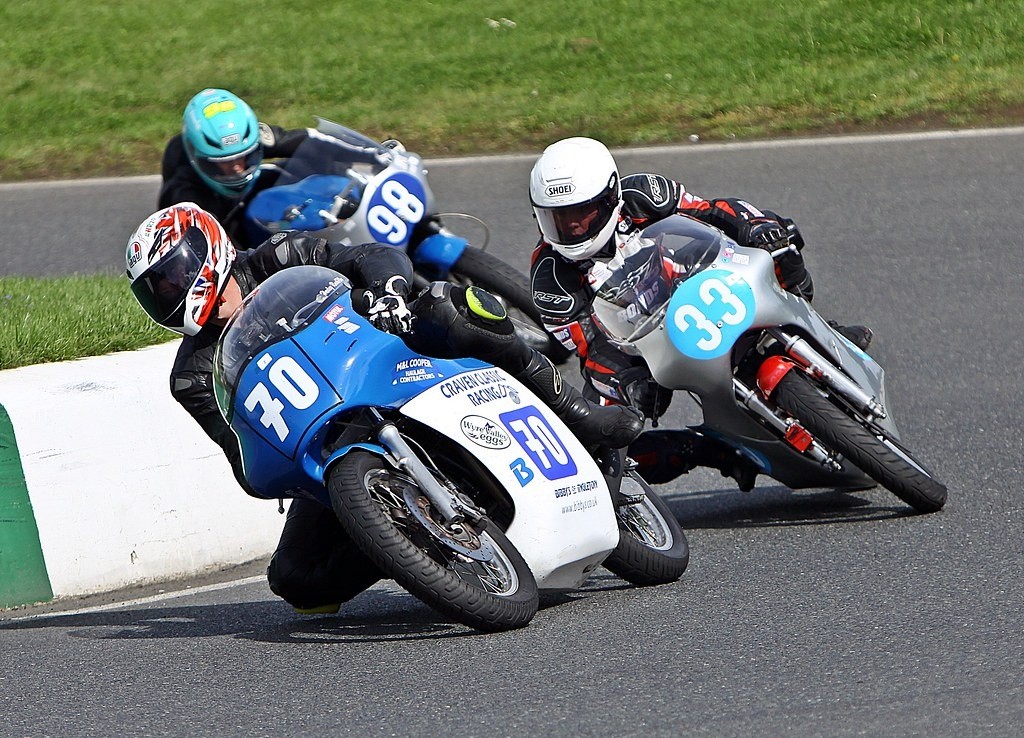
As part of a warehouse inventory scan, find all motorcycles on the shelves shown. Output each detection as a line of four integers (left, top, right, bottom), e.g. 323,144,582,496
585,211,948,514
210,262,690,629
246,114,576,366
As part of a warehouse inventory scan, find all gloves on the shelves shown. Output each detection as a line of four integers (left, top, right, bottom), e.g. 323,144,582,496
748,223,789,253
367,275,413,335
373,139,406,170
618,371,673,418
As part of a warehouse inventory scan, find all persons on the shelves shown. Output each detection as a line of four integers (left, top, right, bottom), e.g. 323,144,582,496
159,90,404,257
529,135,873,494
124,201,645,613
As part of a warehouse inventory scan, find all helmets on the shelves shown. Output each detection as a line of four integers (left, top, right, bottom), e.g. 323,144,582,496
125,201,237,337
181,88,262,199
528,136,622,261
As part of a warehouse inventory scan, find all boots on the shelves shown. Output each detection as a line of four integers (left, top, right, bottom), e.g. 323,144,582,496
789,269,873,350
685,430,757,492
516,345,645,450
